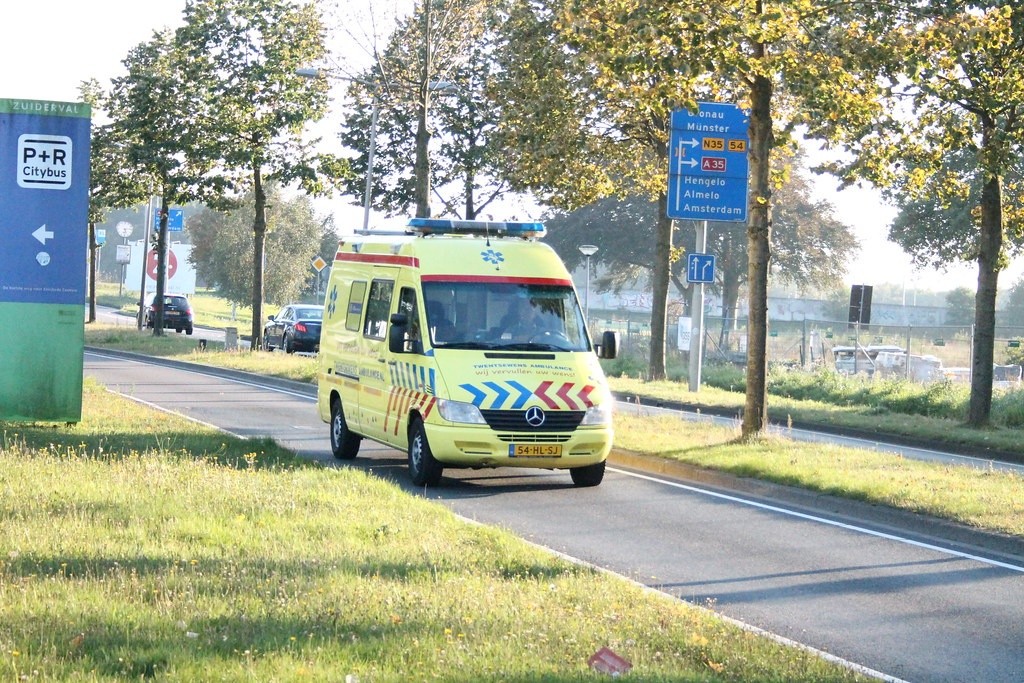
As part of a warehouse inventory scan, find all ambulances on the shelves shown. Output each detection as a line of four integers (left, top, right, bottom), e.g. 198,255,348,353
318,218,619,487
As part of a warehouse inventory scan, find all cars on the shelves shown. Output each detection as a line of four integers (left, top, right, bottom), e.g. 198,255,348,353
136,293,193,335
263,305,324,354
940,368,971,385
627,324,639,333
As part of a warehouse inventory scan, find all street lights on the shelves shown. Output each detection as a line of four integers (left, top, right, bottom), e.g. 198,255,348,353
295,68,452,231
579,244,598,323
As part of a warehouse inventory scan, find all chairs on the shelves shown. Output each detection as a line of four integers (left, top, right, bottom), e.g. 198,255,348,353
500,302,546,332
426,300,458,340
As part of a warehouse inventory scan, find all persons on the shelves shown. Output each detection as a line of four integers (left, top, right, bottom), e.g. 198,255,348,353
501,300,547,341
